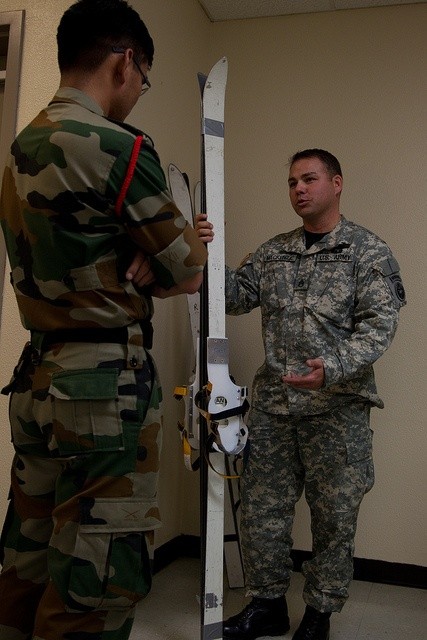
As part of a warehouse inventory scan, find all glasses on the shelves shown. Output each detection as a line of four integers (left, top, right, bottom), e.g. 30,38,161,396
112,47,151,96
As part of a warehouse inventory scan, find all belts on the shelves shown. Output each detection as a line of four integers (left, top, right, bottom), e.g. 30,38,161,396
30,321,154,349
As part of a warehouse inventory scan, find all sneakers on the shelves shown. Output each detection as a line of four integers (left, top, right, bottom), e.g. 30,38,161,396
223,595,290,639
291,604,332,640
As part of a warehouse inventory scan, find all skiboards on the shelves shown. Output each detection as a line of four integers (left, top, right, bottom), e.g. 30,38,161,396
172,55,250,640
168,163,246,588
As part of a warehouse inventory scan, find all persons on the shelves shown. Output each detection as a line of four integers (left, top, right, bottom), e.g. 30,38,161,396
198,148,407,640
0,0,209,640
